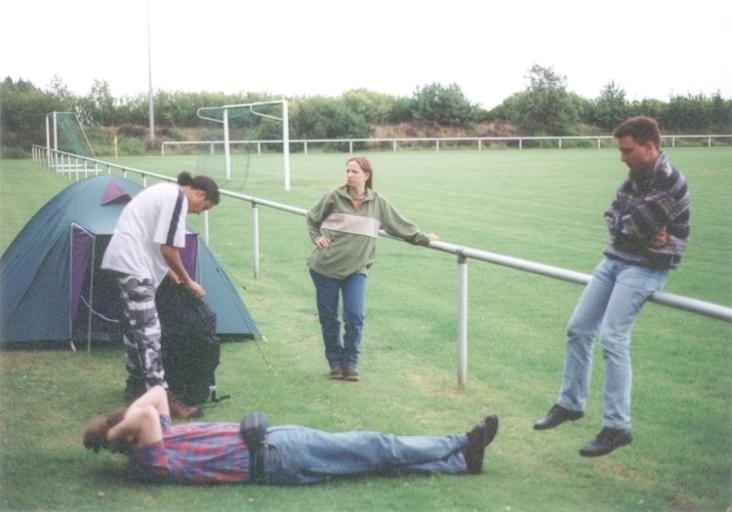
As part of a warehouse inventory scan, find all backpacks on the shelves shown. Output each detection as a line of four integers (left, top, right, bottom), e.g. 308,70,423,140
156,277,231,407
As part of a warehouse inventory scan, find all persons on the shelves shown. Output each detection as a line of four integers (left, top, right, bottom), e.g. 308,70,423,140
529,116,694,458
303,155,439,382
95,168,223,394
78,384,500,482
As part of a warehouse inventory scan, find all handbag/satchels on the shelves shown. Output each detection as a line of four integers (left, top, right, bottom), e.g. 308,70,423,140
239,410,266,445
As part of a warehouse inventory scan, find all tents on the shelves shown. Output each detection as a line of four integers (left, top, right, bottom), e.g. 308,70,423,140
2,171,264,348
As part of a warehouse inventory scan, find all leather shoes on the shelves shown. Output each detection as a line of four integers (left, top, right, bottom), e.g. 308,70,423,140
467,414,499,448
579,427,633,457
330,364,344,379
344,366,360,380
460,421,487,475
532,404,584,430
